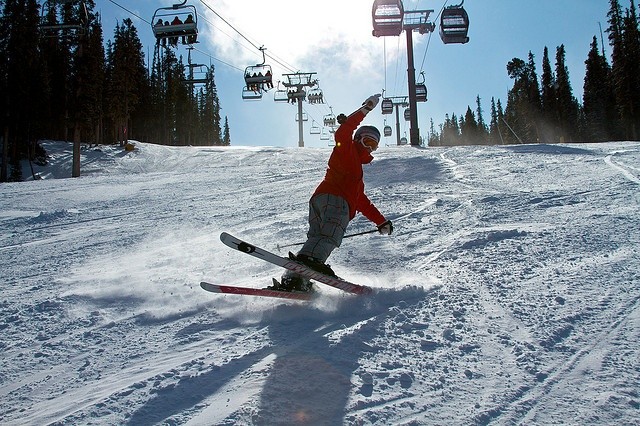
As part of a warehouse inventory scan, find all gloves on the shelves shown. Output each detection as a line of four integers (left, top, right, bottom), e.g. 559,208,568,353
362,93,381,110
377,220,393,235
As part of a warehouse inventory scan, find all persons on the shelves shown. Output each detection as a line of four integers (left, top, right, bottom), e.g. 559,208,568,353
287,89,299,103
244,69,273,92
308,92,324,105
324,117,336,126
154,12,199,47
273,93,393,294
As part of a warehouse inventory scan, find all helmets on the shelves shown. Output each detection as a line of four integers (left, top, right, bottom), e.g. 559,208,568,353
354,126,380,154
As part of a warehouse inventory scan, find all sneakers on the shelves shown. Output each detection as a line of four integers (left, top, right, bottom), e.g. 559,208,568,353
281,270,315,293
297,254,335,277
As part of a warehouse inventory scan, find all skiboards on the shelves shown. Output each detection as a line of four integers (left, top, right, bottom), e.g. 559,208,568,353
200,232,374,299
